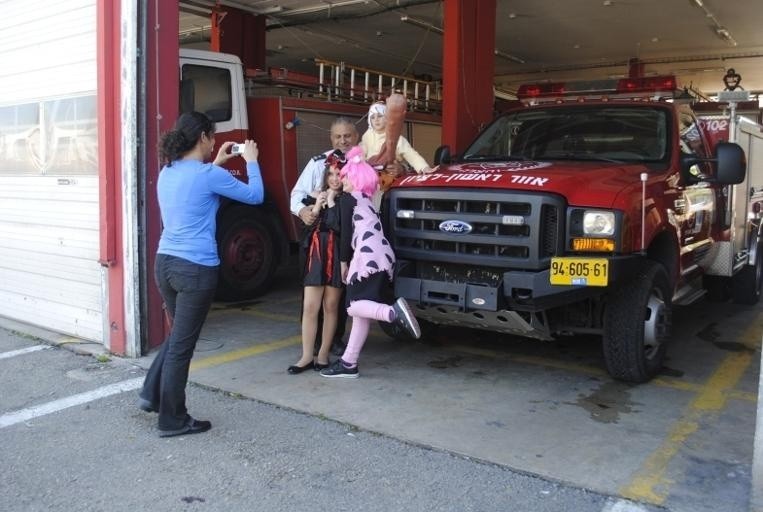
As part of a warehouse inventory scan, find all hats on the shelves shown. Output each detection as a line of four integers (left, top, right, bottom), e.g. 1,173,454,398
367,103,387,131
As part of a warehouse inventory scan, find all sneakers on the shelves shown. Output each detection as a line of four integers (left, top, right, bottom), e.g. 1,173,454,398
391,296,422,340
319,358,360,379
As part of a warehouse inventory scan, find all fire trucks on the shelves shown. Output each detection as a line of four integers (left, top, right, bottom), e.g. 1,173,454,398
361,76,762,384
179,48,441,300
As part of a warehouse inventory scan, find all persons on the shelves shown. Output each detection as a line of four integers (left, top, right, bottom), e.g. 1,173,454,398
135,110,266,439
286,148,356,374
320,146,422,379
289,116,359,225
358,103,439,213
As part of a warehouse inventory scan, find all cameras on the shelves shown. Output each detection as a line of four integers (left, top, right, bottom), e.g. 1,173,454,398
231,144,246,154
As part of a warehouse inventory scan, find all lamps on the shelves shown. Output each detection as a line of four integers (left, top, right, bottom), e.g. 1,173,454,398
400,15,444,38
495,49,525,65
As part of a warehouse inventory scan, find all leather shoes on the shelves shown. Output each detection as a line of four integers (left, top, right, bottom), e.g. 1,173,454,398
312,343,320,355
138,396,160,413
287,358,314,374
330,340,346,356
315,357,331,370
156,415,212,439
